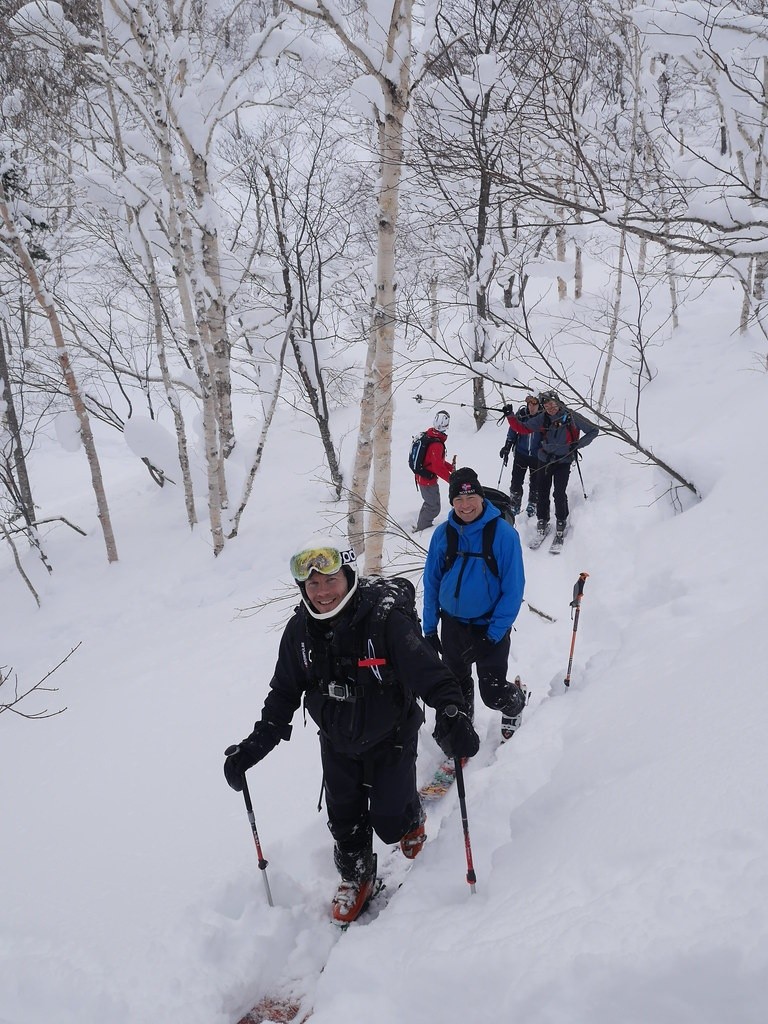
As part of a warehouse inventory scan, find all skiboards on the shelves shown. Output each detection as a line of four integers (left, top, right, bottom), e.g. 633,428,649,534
418,753,472,810
529,518,572,555
238,873,386,1024
514,507,535,518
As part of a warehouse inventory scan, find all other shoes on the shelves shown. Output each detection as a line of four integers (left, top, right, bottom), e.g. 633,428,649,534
556,518,566,531
527,501,537,513
537,519,548,530
509,495,522,515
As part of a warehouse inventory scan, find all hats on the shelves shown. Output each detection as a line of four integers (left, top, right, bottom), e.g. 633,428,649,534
525,396,539,405
449,467,484,506
540,390,560,404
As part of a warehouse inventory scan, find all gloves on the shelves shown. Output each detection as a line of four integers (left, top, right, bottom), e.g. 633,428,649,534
571,441,578,450
503,404,513,416
455,632,491,666
432,709,479,760
424,633,443,657
500,441,513,458
224,719,280,792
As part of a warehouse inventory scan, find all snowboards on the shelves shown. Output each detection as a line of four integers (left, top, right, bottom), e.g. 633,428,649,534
409,525,420,534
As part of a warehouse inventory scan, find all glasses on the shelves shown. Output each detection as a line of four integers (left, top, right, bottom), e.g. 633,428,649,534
289,547,342,580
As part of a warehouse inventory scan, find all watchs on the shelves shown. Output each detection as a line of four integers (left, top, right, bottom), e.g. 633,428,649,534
440,704,468,717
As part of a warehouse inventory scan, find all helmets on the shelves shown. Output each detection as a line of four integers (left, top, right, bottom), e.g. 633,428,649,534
433,410,450,432
290,534,359,593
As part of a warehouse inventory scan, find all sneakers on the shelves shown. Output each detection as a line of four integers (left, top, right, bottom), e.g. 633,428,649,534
334,876,373,923
401,810,426,859
501,675,527,739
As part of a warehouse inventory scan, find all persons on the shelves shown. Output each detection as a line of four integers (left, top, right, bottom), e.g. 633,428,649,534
224,535,480,923
502,391,599,543
499,396,544,517
423,467,525,774
408,411,456,533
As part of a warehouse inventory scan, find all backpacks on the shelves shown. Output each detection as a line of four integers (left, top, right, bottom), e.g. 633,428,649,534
298,574,422,703
443,486,516,578
409,431,435,480
